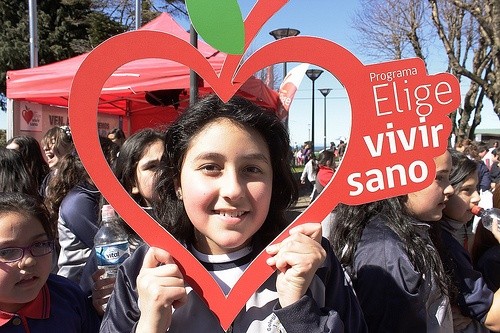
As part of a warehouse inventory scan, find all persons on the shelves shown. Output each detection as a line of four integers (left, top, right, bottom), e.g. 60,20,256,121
0,94,500,332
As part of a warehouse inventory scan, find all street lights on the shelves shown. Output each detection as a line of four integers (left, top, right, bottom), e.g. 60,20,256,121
317,88,333,153
267,27,301,129
304,69,322,151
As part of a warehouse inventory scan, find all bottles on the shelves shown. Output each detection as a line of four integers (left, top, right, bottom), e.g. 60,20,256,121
471,205,500,229
93,206,131,313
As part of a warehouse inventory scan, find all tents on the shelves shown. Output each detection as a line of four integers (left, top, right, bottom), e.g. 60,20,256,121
5,11,288,142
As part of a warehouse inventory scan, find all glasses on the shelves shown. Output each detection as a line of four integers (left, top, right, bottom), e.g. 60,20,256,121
0,240,55,263
